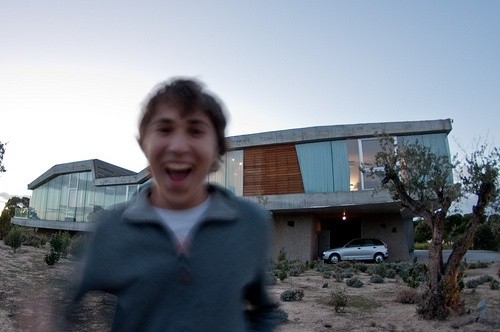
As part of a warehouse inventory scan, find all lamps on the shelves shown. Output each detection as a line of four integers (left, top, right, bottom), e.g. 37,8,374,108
342,209,347,220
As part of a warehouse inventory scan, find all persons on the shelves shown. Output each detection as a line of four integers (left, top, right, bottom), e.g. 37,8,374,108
42,79,284,332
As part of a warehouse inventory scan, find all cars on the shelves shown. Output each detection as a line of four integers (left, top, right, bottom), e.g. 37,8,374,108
322,238,389,263
